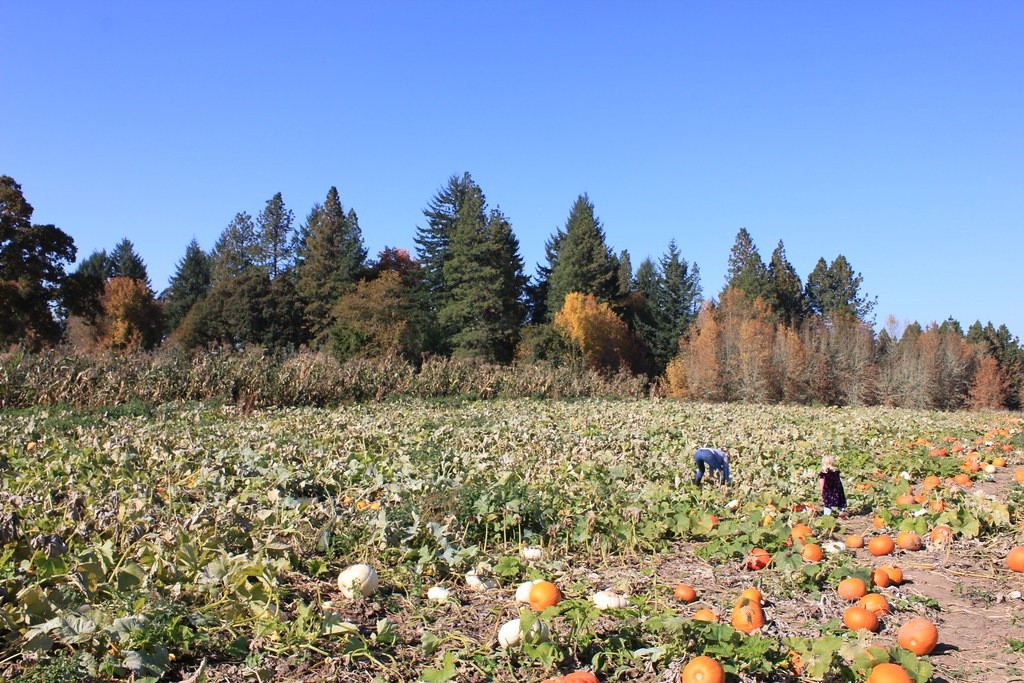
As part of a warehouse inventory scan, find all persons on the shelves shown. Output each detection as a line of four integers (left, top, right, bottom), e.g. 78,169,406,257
818,456,847,516
694,448,731,487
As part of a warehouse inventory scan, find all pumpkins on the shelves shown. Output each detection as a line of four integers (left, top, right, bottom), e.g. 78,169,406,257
339,428,1024,683
